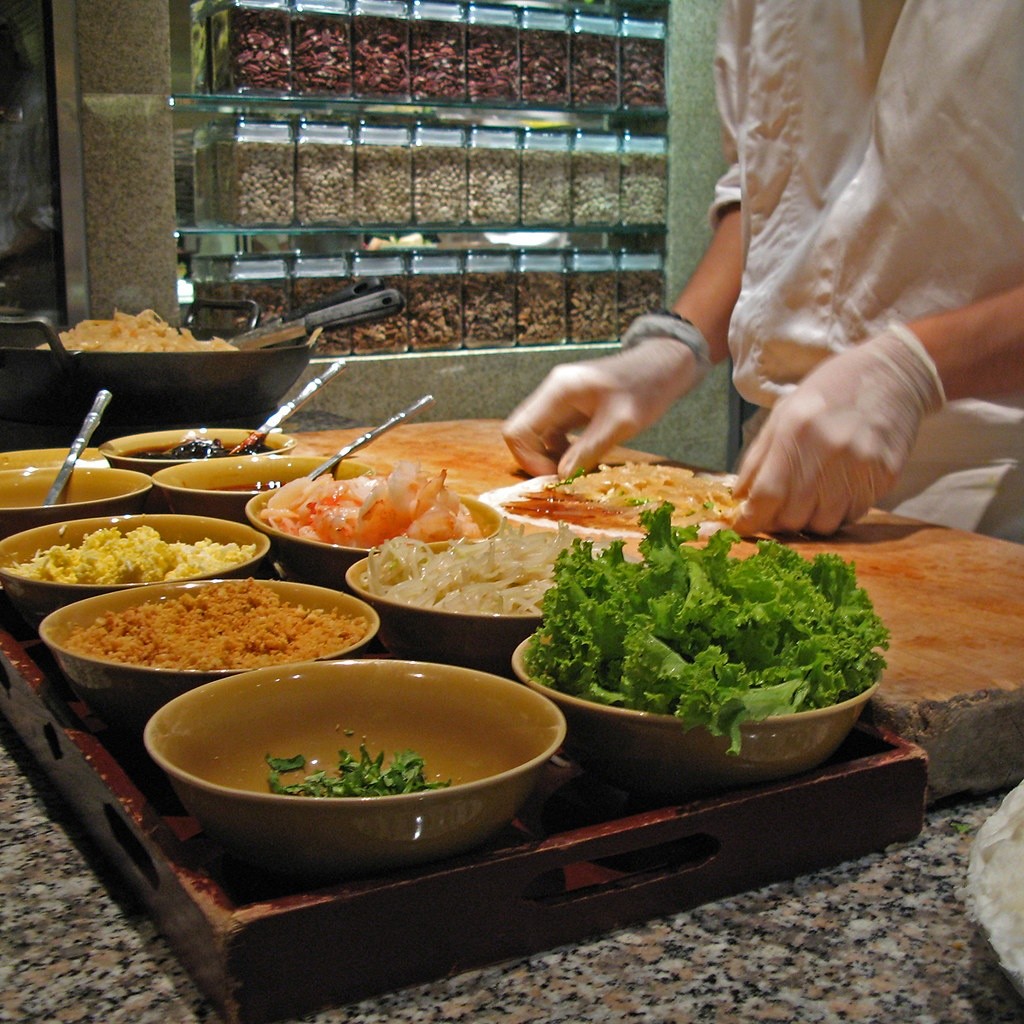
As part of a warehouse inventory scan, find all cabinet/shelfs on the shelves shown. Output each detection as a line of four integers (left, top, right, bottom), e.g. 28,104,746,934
170,95,669,258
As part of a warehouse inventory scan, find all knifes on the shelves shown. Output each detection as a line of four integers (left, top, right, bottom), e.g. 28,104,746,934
226,276,405,352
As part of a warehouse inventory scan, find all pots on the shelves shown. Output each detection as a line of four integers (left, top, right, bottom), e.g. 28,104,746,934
0,300,315,453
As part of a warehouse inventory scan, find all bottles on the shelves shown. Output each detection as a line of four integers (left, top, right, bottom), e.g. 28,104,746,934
190,250,668,357
188,0,669,112
192,113,670,226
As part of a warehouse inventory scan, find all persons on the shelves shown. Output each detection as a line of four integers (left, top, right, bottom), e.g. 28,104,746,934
503,0,1024,544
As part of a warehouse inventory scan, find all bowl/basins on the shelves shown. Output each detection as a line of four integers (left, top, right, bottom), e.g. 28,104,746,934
0,469,153,542
143,660,568,879
152,454,390,529
0,448,111,471
245,492,502,603
345,551,545,687
512,629,883,799
0,514,271,631
38,580,380,733
99,428,299,477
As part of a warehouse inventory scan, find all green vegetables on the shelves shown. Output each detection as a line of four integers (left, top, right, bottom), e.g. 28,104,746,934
265,731,453,799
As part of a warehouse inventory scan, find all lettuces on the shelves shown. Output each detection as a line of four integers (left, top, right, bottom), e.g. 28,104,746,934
524,502,890,759
